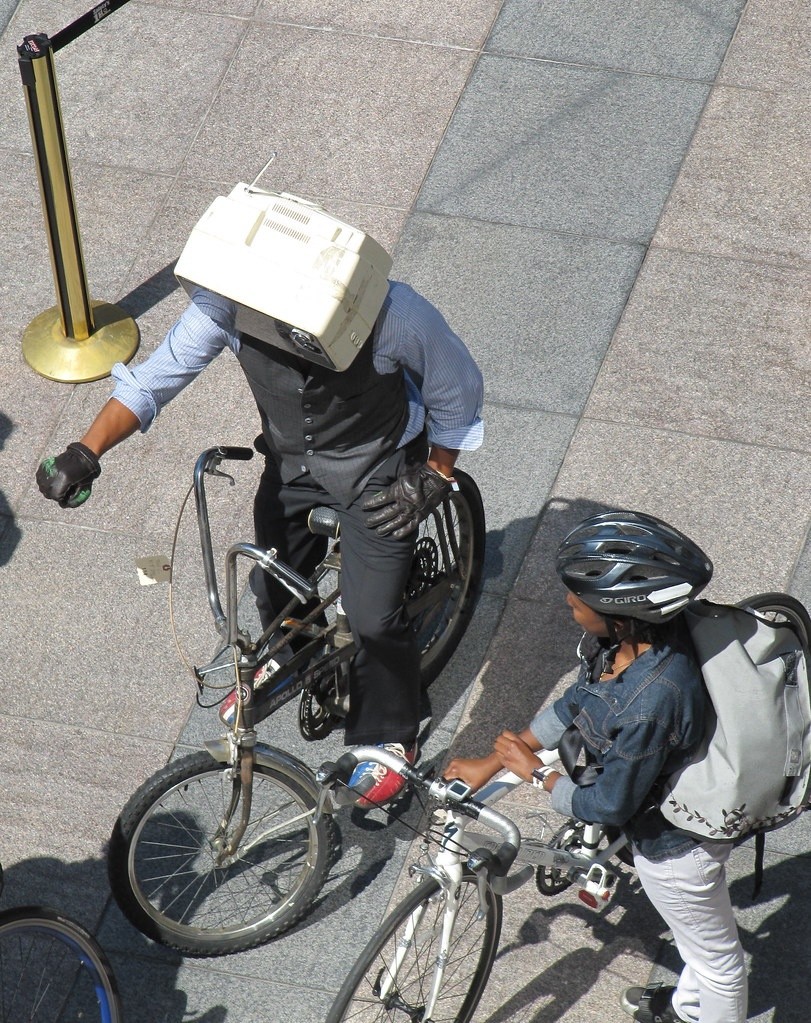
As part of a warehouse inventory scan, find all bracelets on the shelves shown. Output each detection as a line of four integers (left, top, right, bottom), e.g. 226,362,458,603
531,765,559,792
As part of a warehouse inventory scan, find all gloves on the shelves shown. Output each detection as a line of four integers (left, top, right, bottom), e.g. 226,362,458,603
35,441,102,508
361,455,453,541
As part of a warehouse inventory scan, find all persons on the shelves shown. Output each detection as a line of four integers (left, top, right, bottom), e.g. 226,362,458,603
443,510,749,1023
36,182,485,808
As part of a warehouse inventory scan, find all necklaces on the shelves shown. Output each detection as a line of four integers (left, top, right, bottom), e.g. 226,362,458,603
600,644,651,680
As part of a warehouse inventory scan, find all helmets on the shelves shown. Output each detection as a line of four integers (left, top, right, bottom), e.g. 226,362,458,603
555,511,713,622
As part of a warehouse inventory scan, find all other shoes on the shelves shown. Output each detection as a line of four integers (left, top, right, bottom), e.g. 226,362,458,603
621,981,686,1023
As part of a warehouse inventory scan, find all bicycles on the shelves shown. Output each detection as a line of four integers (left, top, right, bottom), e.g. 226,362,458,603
312,592,810,1023
104,445,486,961
0,866,123,1023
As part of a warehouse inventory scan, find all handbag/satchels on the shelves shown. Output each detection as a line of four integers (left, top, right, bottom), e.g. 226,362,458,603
653,600,811,844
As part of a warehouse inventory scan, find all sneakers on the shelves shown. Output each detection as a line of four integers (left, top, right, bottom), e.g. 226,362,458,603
348,738,419,809
219,659,293,729
